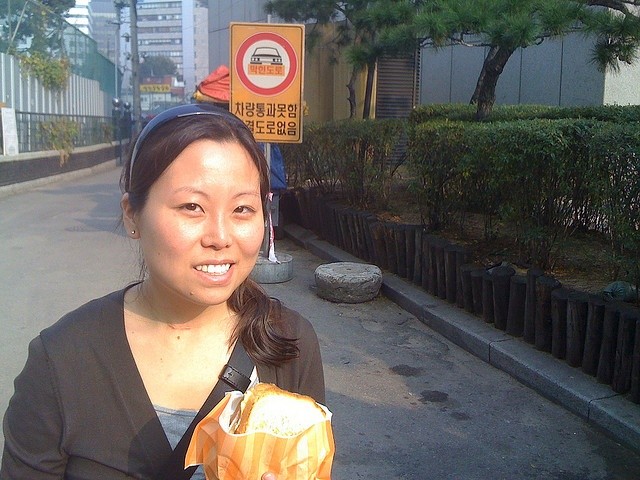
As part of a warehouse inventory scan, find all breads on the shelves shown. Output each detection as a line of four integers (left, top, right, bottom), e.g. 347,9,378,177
231,384,326,438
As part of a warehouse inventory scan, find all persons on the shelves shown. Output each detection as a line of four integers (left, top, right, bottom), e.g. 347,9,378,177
1,103,337,480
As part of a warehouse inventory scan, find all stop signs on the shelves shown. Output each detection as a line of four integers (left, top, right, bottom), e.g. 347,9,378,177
228,22,305,143
